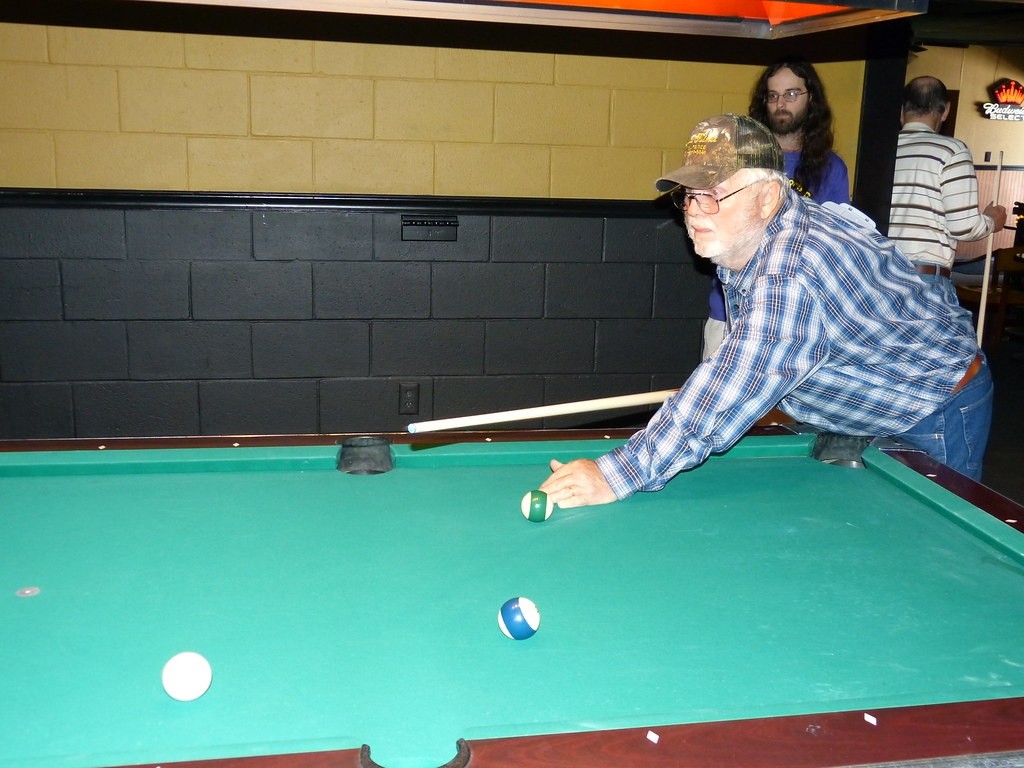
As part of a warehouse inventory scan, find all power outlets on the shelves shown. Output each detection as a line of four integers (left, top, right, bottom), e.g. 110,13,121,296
399,382,419,415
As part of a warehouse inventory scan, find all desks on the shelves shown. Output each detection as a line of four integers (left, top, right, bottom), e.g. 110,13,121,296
0,421,1024,768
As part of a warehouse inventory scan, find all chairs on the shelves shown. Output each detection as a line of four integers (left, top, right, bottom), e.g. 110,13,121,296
956,247,1024,352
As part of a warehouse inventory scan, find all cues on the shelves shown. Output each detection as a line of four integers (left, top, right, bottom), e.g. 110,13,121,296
406,388,681,435
976,150,1004,348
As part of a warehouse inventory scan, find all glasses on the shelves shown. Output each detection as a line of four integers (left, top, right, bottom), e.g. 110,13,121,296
766,91,809,103
670,178,766,214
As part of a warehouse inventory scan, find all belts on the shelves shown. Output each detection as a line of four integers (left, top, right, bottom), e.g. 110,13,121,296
915,265,951,277
951,352,983,395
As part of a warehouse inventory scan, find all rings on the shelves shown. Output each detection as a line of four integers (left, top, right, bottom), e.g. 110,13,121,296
567,486,576,498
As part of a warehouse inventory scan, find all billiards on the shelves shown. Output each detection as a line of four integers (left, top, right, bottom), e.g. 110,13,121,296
498,596,541,640
521,490,554,522
162,652,213,703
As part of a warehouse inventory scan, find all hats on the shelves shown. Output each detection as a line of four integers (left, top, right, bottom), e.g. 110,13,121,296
655,113,785,192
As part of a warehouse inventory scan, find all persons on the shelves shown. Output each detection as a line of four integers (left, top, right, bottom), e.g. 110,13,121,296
701,59,851,362
887,75,1007,306
538,113,995,509
